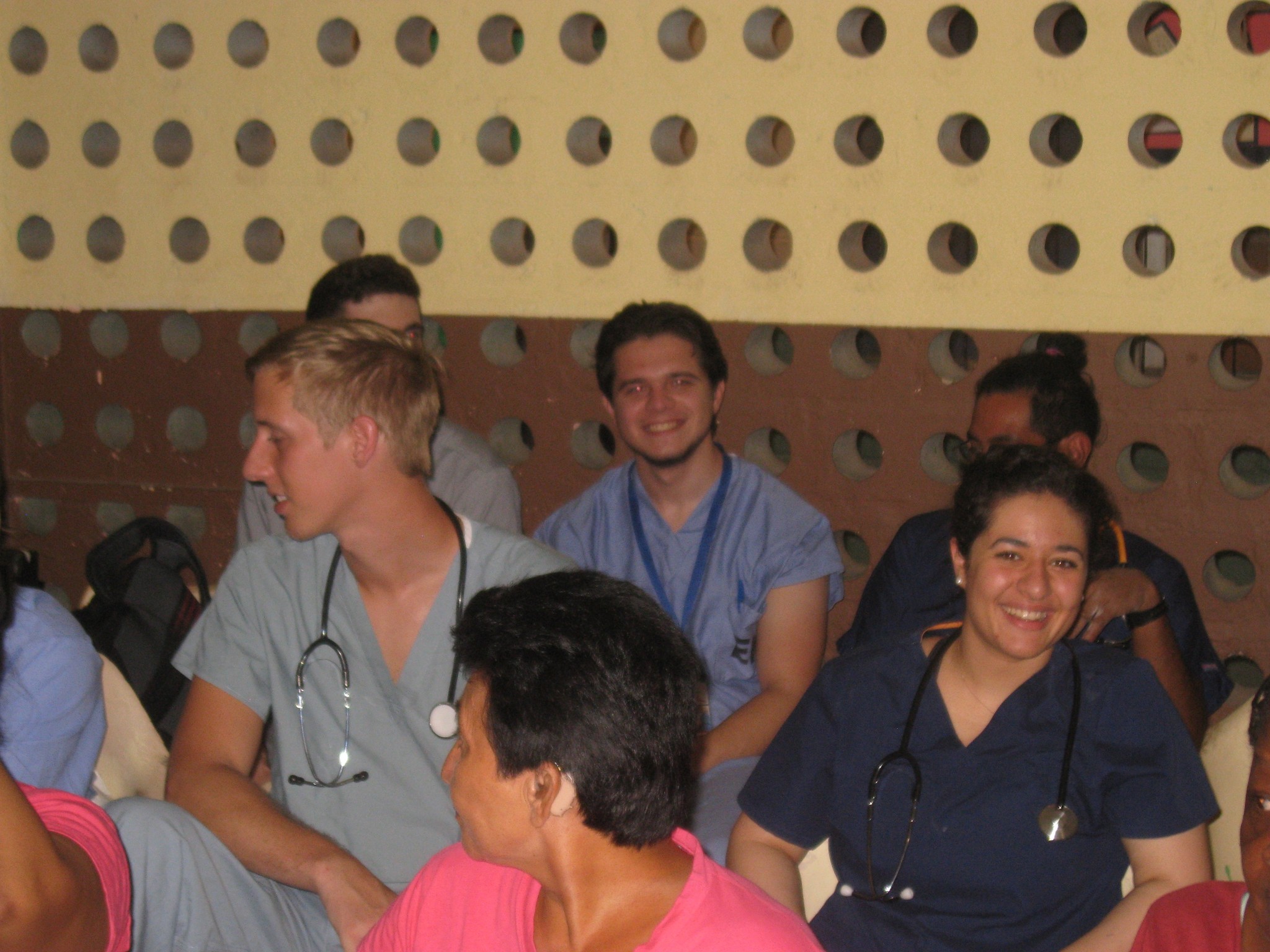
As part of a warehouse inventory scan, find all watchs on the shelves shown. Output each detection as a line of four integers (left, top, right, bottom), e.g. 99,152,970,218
1122,594,1167,631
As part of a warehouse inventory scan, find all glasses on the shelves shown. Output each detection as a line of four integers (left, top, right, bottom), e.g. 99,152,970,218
959,439,1003,461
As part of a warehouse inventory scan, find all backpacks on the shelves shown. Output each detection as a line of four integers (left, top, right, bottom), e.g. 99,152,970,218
83,518,209,744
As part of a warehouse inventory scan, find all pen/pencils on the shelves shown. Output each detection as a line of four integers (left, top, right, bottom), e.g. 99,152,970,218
737,579,744,614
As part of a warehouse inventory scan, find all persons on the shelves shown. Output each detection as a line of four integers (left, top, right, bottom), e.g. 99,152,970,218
1130,676,1269,951
0,470,107,798
0,758,133,952
101,318,584,952
236,254,521,546
836,350,1233,758
354,565,826,951
725,443,1222,952
527,303,841,866
